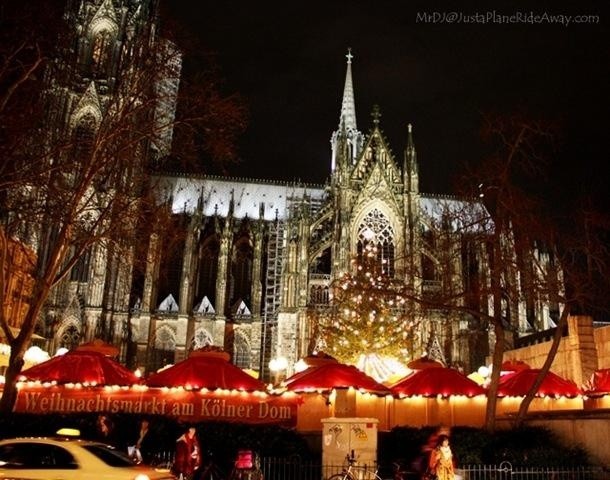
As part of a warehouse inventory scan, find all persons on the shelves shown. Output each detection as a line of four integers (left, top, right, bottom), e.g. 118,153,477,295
171,424,202,480
420,426,449,480
429,433,455,480
126,418,154,462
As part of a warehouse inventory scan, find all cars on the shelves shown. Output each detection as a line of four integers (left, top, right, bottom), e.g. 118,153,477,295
0,435,177,480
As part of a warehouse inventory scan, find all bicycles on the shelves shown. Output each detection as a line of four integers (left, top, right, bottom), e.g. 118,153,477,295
327,451,404,480
